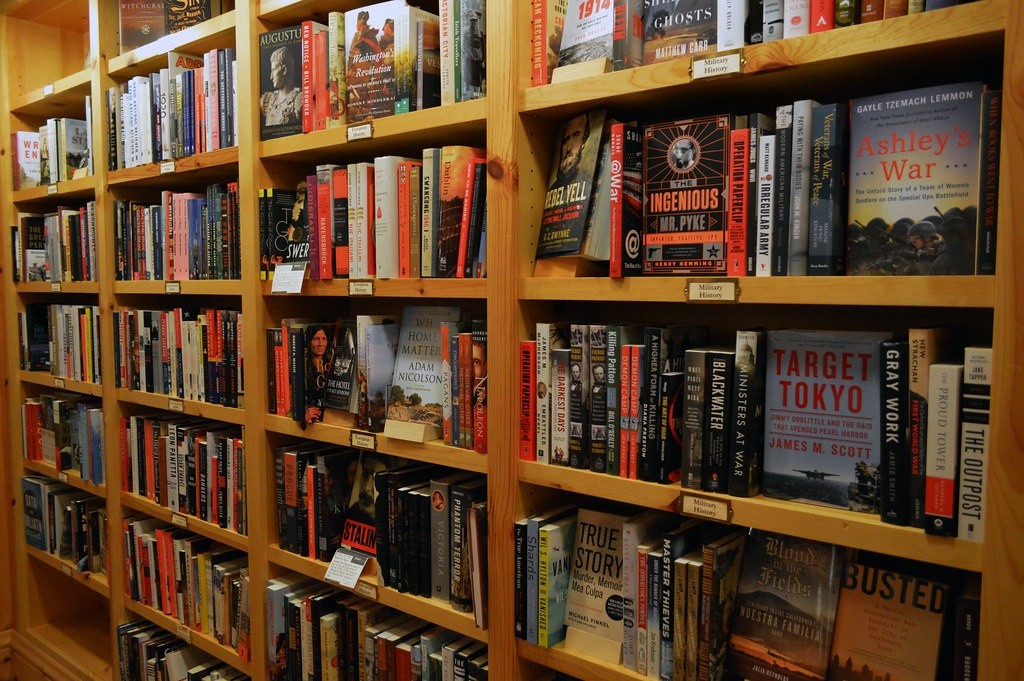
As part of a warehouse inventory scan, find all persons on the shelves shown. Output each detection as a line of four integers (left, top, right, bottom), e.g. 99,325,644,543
461,11,487,101
432,491,444,510
674,140,697,169
324,464,335,520
550,112,593,188
591,363,606,396
385,20,394,49
570,362,582,394
348,10,378,69
345,456,359,489
347,448,400,525
304,325,332,426
378,18,391,52
260,47,305,128
472,338,487,407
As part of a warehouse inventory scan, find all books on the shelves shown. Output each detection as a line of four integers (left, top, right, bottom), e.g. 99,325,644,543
514,0,1006,681
8,0,487,681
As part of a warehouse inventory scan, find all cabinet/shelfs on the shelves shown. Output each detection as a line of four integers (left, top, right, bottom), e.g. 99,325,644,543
0,0,1024,681
